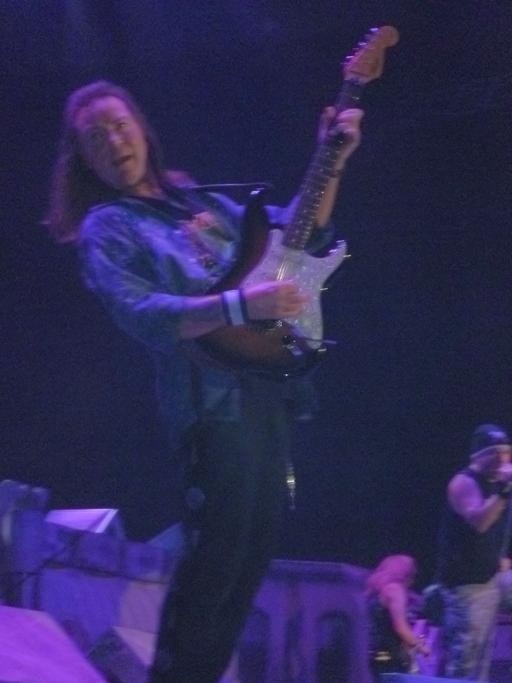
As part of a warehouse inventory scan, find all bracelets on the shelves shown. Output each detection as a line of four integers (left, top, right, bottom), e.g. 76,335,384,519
221,287,248,328
331,166,347,180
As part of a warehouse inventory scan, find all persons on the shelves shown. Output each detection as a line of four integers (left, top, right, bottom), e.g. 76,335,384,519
443,424,512,683
38,78,367,683
362,555,431,683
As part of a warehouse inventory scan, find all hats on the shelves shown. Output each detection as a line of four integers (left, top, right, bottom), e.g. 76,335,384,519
465,424,510,459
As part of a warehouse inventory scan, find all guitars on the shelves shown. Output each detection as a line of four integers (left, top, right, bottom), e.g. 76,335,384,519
176,23,400,378
